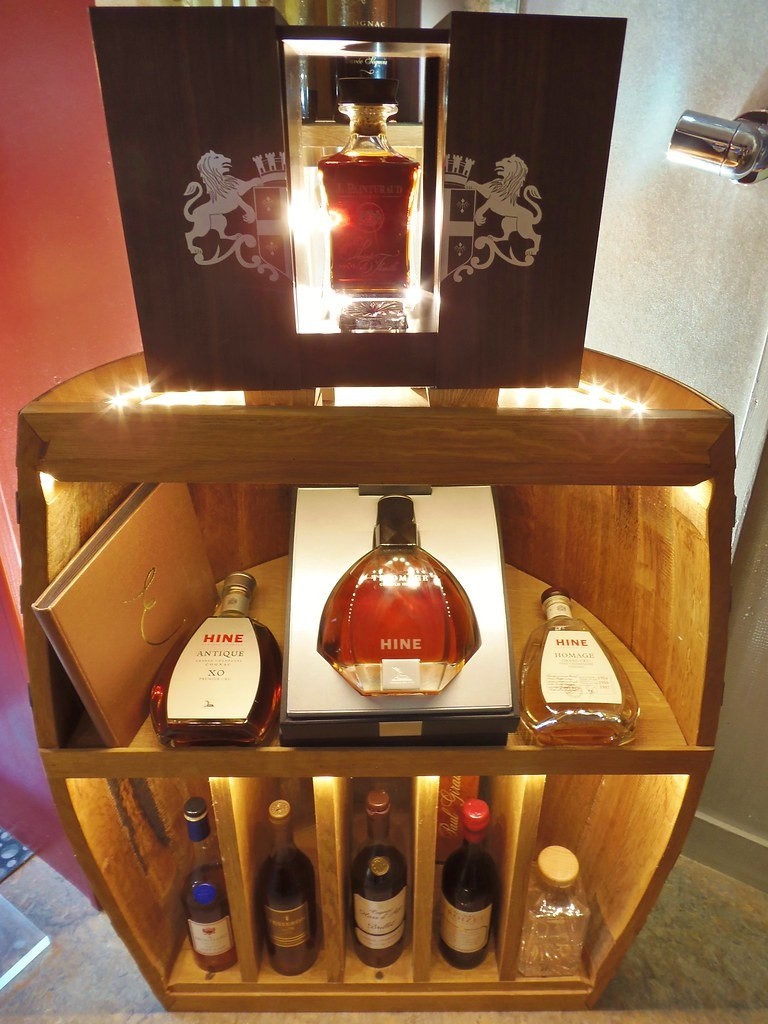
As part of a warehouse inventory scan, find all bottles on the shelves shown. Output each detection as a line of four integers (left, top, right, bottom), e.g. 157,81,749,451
350,789,408,968
258,800,318,977
148,571,285,746
517,845,591,979
436,799,498,970
315,75,422,333
318,492,483,698
182,796,237,972
515,586,642,747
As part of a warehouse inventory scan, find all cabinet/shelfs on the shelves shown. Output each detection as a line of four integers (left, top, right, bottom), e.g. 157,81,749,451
18,348,739,1008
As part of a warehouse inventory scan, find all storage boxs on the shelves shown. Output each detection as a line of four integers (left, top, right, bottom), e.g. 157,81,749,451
278,484,521,746
